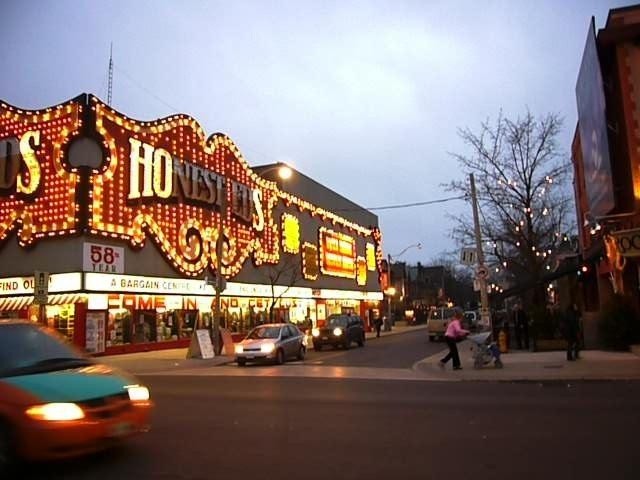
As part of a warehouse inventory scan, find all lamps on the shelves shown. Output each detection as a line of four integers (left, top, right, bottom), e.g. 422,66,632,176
583,211,600,236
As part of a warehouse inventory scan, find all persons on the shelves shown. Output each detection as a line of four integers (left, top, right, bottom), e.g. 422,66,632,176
564,301,584,361
374,314,383,337
510,304,530,350
303,315,313,336
437,313,471,370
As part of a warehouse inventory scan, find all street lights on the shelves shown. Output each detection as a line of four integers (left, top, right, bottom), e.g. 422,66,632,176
387,242,422,331
213,166,293,356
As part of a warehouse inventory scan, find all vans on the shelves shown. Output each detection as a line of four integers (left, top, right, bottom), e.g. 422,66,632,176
463,311,481,333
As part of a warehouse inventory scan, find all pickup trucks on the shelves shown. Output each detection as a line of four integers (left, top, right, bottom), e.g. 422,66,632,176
426,306,466,342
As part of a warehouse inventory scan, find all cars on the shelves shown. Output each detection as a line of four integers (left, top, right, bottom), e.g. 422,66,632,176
0,316,155,462
233,323,308,367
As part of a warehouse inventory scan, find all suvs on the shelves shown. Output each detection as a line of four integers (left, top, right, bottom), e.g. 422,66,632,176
312,311,366,352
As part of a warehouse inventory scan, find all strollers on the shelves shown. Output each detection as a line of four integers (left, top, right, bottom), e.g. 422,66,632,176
466,330,504,370
